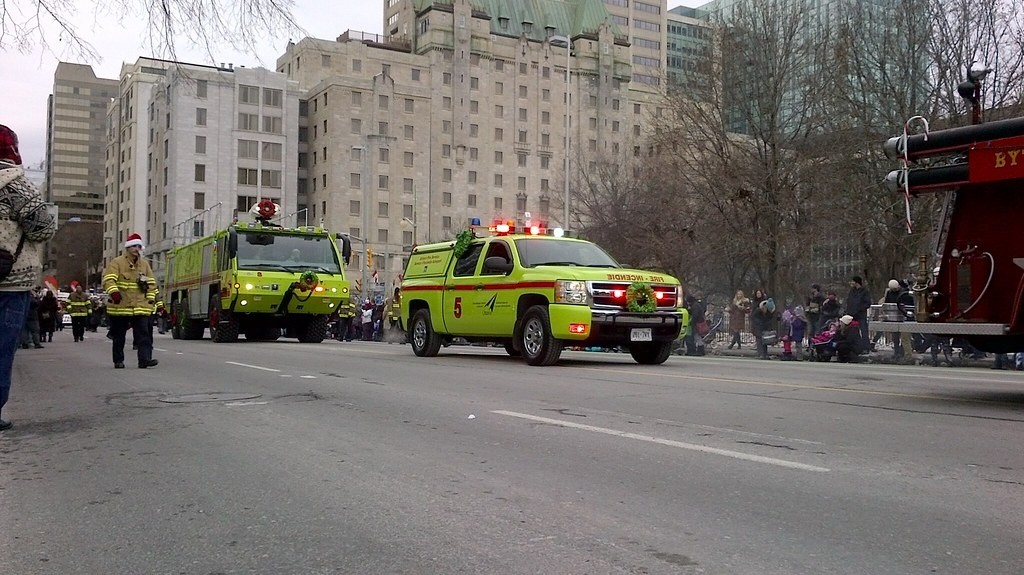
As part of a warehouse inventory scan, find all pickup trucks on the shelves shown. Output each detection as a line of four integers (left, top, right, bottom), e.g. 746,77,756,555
395,220,689,367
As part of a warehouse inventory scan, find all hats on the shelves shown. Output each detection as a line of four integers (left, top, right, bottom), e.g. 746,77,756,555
0,124,22,165
765,298,775,309
853,276,862,286
840,315,853,324
124,233,145,250
795,305,805,316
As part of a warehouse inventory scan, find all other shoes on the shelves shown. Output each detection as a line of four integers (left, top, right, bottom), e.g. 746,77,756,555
35,344,45,348
138,359,158,368
737,344,741,349
60,329,62,331
40,336,46,342
56,329,58,331
159,331,165,334
337,337,352,342
48,336,52,342
22,344,28,349
870,343,878,352
728,342,736,349
80,337,83,341
114,362,125,368
0,420,13,431
74,338,78,342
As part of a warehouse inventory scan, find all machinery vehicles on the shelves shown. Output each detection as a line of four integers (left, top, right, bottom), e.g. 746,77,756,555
162,211,351,343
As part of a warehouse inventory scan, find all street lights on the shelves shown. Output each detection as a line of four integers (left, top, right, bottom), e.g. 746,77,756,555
69,254,89,293
549,33,571,230
342,234,367,303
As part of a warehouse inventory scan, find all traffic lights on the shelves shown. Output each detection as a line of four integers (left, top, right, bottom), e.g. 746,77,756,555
366,248,372,267
355,279,369,299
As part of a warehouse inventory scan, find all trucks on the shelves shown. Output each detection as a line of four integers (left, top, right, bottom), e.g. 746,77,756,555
41,288,72,324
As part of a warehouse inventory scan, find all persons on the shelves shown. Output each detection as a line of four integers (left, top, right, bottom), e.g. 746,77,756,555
253,246,272,260
103,234,158,368
22,283,171,350
683,295,704,356
0,125,55,435
725,290,750,349
779,284,862,363
870,279,913,352
845,276,871,354
752,289,765,310
751,298,780,359
286,248,301,262
331,295,386,341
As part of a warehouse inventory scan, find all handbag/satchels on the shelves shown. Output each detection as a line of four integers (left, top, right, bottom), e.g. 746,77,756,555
696,321,709,334
0,248,13,282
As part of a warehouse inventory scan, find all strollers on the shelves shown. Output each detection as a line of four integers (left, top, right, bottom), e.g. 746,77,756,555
809,318,839,363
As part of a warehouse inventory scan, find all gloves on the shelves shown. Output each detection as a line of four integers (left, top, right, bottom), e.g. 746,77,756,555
111,292,123,304
